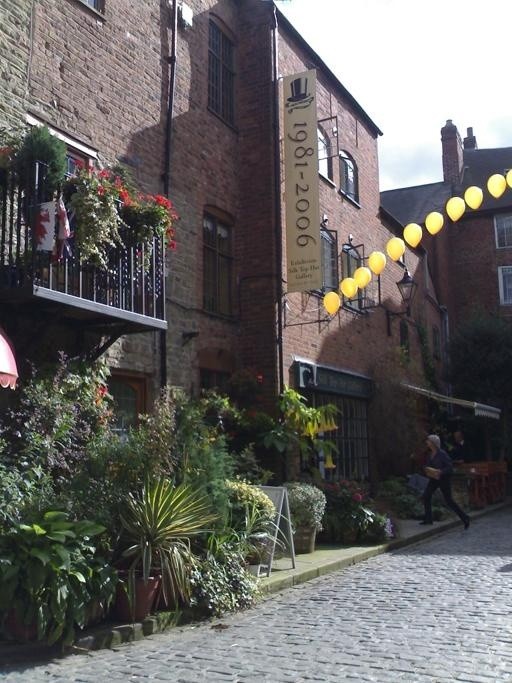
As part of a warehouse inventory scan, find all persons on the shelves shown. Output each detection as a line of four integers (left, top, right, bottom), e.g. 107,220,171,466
450,430,472,464
418,435,470,529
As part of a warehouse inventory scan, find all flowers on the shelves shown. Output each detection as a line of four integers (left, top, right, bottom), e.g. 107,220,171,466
325,476,396,542
63,160,129,274
131,193,180,270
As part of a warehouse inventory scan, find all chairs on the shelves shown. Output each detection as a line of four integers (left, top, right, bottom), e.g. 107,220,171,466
463,461,507,509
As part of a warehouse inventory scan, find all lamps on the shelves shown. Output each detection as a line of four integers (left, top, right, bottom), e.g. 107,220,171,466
386,267,420,337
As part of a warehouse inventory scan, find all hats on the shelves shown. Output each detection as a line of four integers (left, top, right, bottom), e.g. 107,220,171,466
426,434,442,448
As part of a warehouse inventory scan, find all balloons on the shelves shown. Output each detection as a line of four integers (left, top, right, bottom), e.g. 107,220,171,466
323,291,340,316
445,196,465,222
464,185,484,210
353,266,372,287
385,237,405,262
403,223,423,248
506,169,512,189
487,175,506,198
426,211,443,237
368,251,386,274
338,277,357,298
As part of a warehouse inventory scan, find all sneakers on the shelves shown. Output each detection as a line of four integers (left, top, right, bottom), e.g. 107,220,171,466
419,516,470,531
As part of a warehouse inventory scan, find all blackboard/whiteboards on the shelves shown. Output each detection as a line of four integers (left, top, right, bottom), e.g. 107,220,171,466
257,486,285,565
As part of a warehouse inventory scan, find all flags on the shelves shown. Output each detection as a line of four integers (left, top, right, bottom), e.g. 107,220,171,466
35,199,76,265
